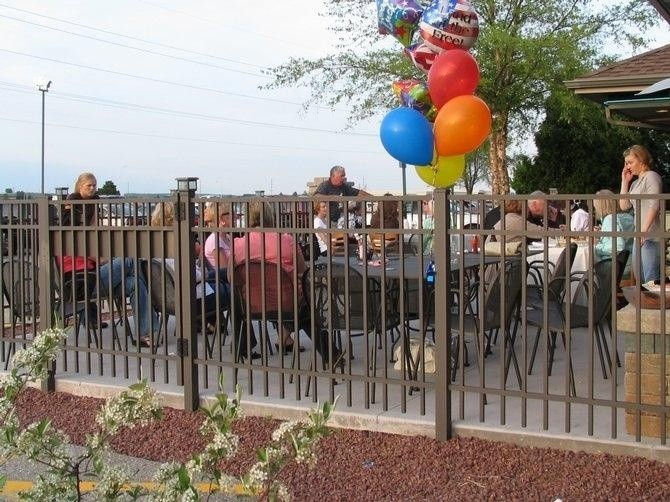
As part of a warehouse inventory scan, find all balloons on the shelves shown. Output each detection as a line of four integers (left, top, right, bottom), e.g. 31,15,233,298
375,1,493,191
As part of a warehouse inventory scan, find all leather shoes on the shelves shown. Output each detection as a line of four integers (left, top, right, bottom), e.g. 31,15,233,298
275,343,306,354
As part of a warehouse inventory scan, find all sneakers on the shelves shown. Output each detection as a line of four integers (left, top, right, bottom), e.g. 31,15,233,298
85,320,108,329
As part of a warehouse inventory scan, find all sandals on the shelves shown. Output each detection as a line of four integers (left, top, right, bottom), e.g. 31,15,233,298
133,339,159,347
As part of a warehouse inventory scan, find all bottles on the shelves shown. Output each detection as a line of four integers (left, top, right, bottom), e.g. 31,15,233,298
471,235,479,256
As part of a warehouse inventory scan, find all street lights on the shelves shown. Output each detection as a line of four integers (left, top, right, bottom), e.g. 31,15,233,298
35,80,51,195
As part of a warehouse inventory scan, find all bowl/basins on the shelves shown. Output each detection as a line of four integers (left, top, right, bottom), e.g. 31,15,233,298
621,285,670,310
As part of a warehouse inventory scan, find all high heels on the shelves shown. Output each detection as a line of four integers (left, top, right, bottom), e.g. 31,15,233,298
240,350,262,360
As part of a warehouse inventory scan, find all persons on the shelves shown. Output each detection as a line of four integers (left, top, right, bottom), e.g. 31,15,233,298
56,173,348,369
618,144,666,284
482,188,636,266
311,166,456,254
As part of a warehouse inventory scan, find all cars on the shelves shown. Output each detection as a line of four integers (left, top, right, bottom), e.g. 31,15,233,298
364,194,496,231
98,193,247,232
